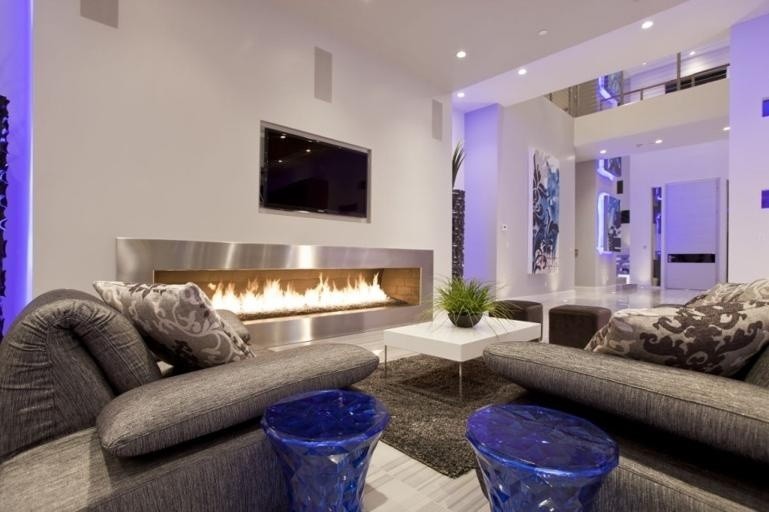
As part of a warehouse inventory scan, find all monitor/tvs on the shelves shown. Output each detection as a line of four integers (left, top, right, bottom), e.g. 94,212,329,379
258,120,371,224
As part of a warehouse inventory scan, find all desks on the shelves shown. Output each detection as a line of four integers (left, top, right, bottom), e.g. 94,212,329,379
265,387,389,512
468,403,620,512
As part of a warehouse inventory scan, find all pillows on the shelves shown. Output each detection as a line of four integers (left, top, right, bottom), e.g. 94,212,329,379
92,280,255,368
583,298,768,375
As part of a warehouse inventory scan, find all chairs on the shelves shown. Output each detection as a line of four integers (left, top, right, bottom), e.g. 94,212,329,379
1,280,379,511
482,279,769,511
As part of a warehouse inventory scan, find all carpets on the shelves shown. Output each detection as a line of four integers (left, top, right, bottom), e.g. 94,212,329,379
349,353,526,479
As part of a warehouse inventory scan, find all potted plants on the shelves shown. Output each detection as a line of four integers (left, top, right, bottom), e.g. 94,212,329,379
452,138,468,277
414,275,521,341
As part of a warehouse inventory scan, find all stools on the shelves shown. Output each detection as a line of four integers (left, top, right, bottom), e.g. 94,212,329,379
548,304,612,348
486,298,545,344
